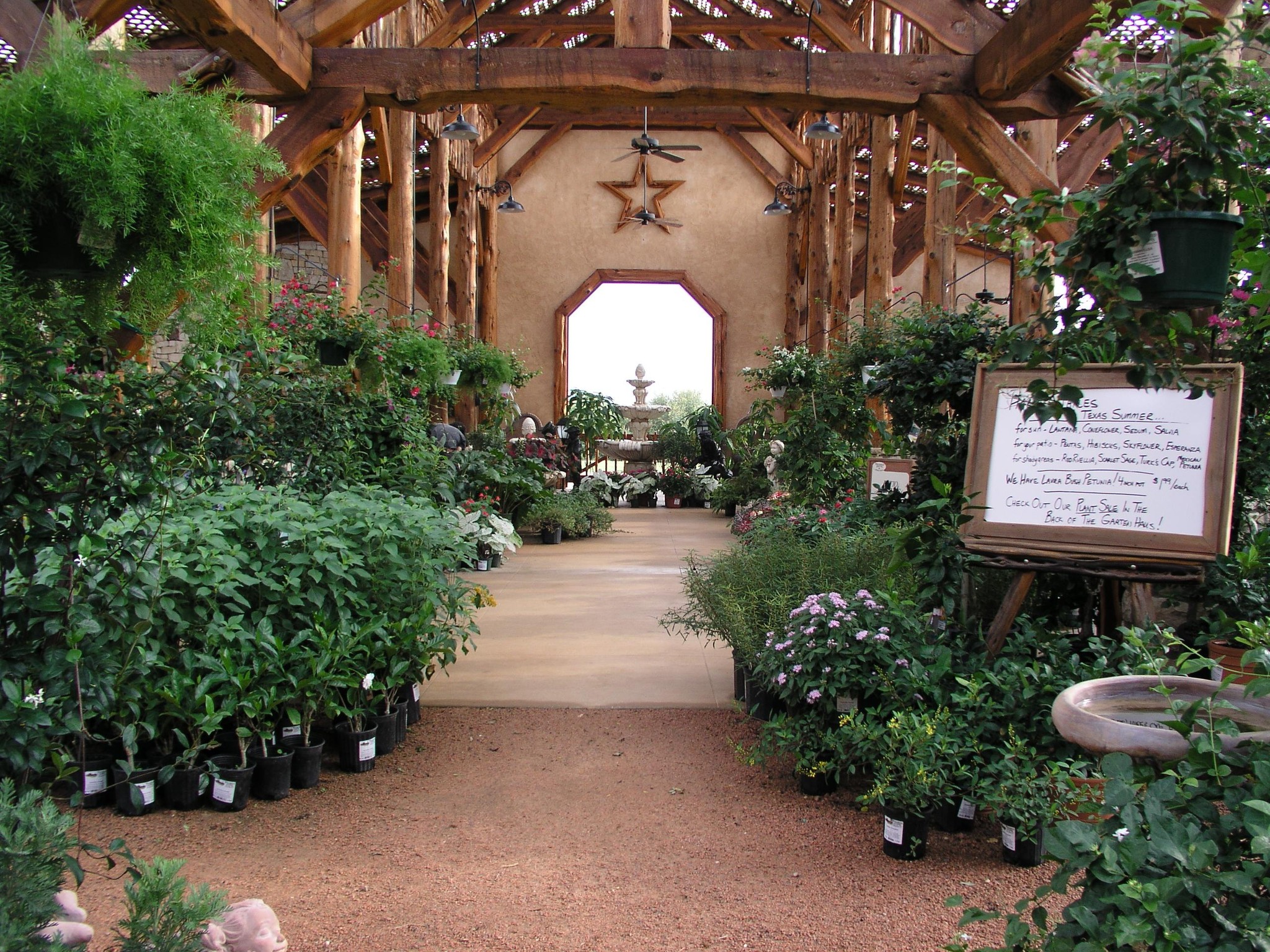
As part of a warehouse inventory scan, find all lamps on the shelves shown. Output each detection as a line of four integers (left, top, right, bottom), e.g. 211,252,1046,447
477,179,524,215
442,103,480,140
764,180,813,215
805,111,843,140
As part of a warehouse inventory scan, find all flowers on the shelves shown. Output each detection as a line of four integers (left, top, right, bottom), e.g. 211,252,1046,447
840,707,963,816
256,274,858,537
751,585,950,704
721,701,859,779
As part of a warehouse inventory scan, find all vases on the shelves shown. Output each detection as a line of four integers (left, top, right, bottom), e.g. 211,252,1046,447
648,499,656,508
801,751,836,794
664,494,682,508
631,499,639,508
883,805,929,862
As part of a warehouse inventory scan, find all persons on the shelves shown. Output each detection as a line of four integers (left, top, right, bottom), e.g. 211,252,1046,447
541,421,581,493
683,430,726,467
764,440,784,490
433,421,466,451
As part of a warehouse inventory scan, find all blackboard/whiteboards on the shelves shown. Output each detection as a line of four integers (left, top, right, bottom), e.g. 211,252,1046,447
960,362,1244,561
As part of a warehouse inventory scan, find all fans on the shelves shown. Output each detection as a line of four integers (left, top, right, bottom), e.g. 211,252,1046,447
607,152,683,231
612,106,702,162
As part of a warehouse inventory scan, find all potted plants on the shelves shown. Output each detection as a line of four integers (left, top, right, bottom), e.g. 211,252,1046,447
1041,0,1269,308
0,457,1270,866
